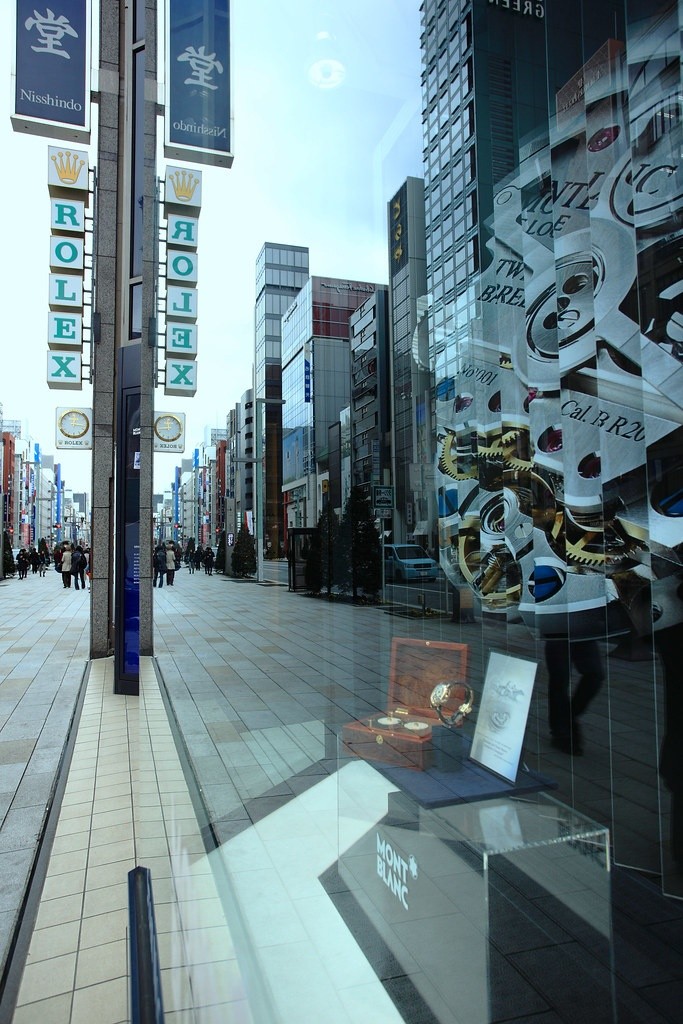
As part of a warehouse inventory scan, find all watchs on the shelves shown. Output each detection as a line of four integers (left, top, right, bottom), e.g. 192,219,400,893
430,679,473,727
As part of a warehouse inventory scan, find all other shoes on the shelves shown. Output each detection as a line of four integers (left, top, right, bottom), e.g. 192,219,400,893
87,585,90,588
75,586,79,590
82,585,85,589
88,589,91,593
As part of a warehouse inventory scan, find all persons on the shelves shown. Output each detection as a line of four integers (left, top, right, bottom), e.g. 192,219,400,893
154,541,286,588
15,538,90,586
540,610,610,759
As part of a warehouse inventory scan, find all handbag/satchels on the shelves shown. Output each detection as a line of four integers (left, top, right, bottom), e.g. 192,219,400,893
174,560,181,571
55,561,63,573
69,560,79,576
156,550,166,563
